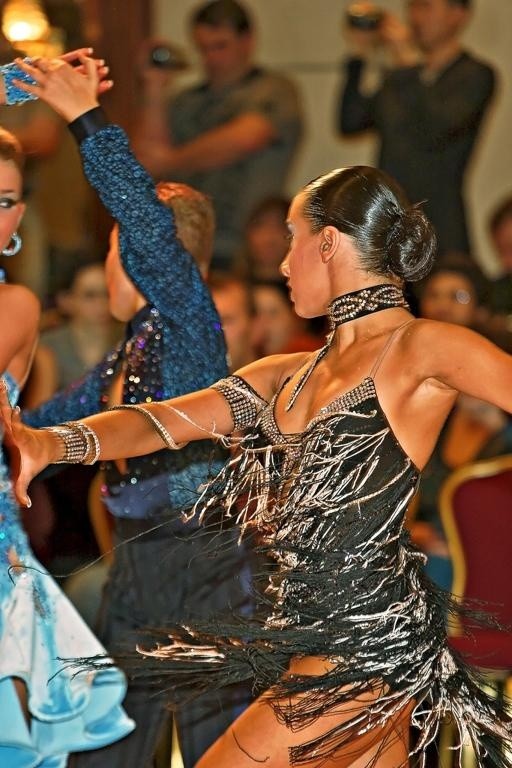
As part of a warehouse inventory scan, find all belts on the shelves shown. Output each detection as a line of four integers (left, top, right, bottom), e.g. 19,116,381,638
104,490,243,544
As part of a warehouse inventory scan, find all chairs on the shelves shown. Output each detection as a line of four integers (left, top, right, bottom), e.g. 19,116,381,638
427,449,511,768
237,483,283,546
16,338,60,414
83,462,119,567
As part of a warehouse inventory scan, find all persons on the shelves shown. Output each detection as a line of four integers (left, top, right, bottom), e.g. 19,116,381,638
0,1,511,767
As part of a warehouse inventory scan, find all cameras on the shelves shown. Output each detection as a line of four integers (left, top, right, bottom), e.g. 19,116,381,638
151,46,183,66
349,11,380,30
